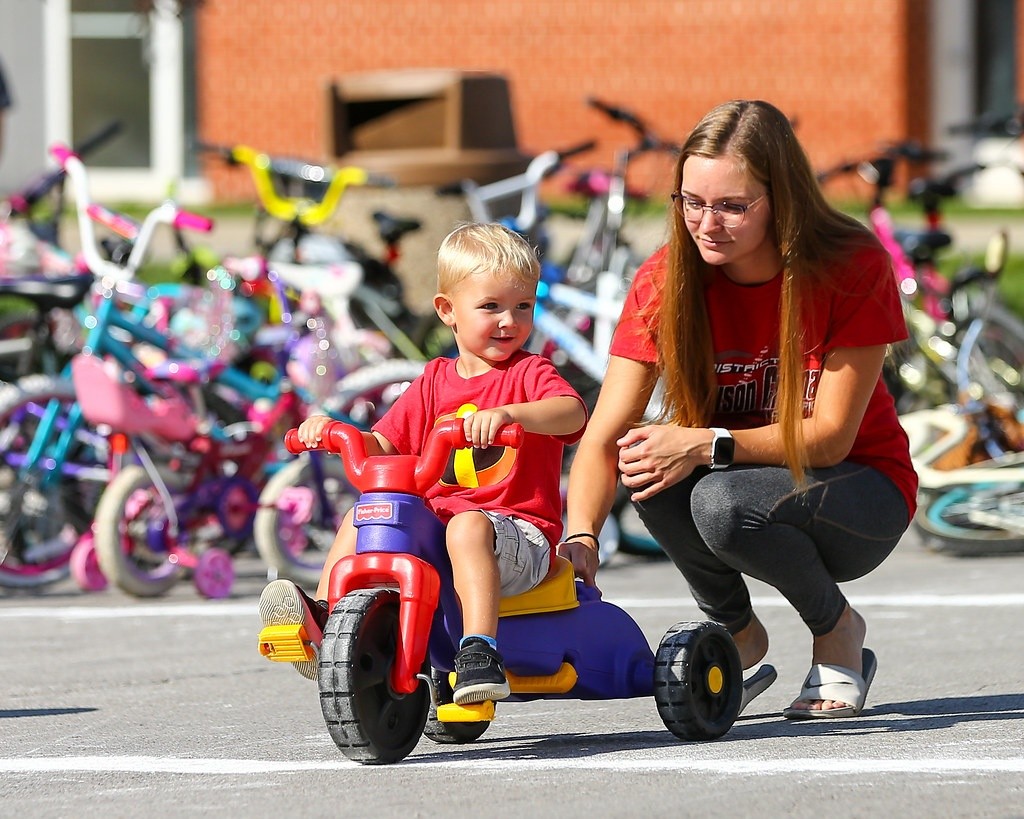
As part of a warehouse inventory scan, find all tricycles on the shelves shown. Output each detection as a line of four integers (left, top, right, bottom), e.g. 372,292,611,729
258,422,743,766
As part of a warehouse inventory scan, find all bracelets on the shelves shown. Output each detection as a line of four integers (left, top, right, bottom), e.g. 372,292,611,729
565,533,599,550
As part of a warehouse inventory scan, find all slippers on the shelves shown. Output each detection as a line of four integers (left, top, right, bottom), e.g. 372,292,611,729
783,648,877,718
740,663,778,712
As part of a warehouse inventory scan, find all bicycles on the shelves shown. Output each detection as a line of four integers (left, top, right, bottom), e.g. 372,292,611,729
1,96,1024,601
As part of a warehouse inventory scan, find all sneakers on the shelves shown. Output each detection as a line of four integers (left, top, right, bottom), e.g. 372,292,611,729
451,636,510,704
258,579,332,681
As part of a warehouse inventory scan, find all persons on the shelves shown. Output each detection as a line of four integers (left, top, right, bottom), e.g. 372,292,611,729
558,99,921,719
258,223,590,707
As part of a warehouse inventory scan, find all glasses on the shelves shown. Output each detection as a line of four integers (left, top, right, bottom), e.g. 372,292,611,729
670,190,769,228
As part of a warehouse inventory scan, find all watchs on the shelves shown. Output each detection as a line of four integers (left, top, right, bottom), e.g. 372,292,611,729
708,428,735,469
